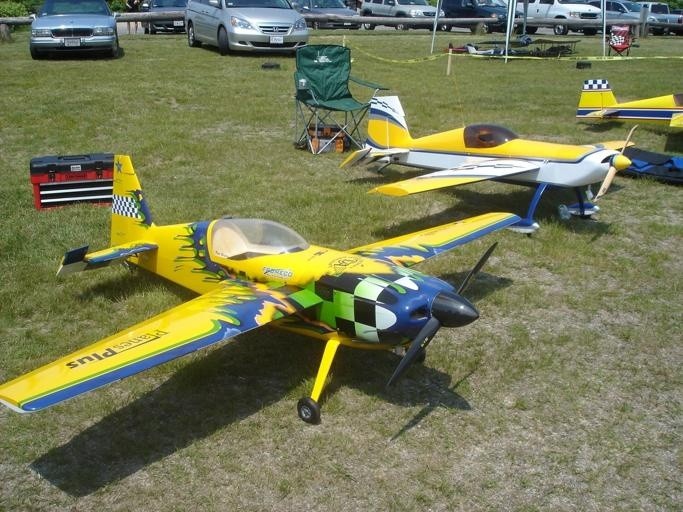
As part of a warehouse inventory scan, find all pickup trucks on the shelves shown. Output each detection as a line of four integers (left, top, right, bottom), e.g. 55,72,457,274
517,0,601,35
636,2,683,35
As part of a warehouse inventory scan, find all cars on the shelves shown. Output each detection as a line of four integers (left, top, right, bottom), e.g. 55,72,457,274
292,0,361,30
140,0,188,34
29,0,120,58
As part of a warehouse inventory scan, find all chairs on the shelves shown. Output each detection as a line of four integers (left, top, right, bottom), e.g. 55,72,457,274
608,25,632,56
294,44,390,155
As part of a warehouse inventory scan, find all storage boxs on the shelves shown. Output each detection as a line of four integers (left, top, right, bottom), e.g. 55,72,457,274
310,125,345,137
310,139,343,154
30,153,115,209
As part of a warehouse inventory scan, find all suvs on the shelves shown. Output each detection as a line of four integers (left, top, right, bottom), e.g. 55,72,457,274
360,0,445,30
440,0,526,34
588,0,659,34
185,0,309,55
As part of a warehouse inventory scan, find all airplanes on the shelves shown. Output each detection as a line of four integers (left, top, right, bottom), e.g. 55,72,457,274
337,97,639,237
0,153,519,424
571,80,683,131
